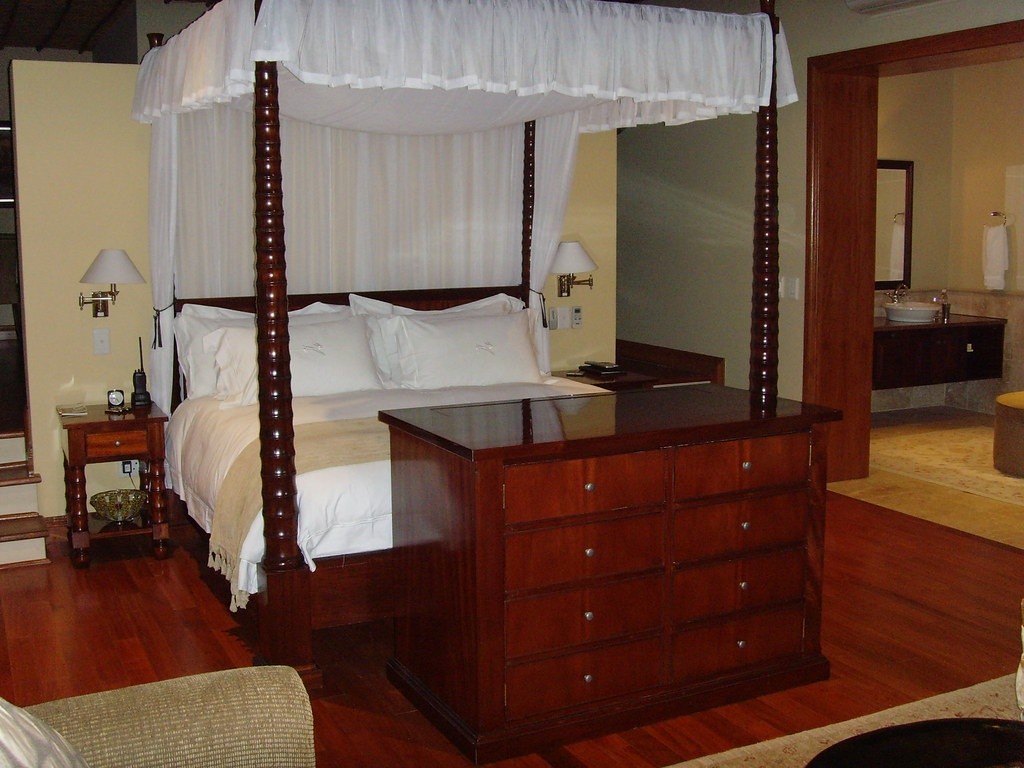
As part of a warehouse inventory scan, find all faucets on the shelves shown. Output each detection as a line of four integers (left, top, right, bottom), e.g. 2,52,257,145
893,284,908,303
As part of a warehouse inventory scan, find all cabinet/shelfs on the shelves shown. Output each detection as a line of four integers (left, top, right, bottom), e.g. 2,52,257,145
375,382,841,768
871,324,1007,390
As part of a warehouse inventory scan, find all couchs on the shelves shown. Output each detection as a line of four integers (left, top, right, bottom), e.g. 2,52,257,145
0,667,317,768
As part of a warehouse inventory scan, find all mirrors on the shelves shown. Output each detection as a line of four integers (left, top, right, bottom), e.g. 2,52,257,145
874,159,915,290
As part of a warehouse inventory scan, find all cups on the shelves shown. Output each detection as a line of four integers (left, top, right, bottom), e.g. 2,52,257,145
942,302,951,320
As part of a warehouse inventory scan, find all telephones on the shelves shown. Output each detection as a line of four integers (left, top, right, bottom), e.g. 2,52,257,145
130,337,152,406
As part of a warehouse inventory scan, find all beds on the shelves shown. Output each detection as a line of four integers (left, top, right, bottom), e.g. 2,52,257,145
133,0,802,692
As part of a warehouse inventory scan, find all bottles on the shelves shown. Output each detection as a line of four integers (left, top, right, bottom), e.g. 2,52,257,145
939,289,948,316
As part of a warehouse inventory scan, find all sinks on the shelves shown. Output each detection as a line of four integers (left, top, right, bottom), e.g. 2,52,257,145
880,302,941,322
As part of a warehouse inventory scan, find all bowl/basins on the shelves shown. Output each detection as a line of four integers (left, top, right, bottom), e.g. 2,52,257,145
89,489,147,521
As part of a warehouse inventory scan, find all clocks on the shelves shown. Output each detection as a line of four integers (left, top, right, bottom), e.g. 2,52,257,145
107,390,126,408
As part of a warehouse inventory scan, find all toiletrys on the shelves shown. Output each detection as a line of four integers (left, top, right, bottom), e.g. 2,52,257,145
941,289,951,319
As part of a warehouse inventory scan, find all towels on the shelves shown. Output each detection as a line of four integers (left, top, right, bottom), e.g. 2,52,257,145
889,224,904,281
981,224,1009,290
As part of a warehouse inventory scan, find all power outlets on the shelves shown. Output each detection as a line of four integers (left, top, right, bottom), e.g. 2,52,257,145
118,459,139,479
558,307,570,331
92,328,112,357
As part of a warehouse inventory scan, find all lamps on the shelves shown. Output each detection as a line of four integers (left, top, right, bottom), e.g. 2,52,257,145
77,250,144,316
549,242,597,297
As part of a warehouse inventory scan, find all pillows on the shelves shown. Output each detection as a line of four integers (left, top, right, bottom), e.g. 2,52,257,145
176,294,545,400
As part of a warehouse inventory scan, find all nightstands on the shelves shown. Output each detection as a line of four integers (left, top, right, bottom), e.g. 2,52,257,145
55,400,170,569
